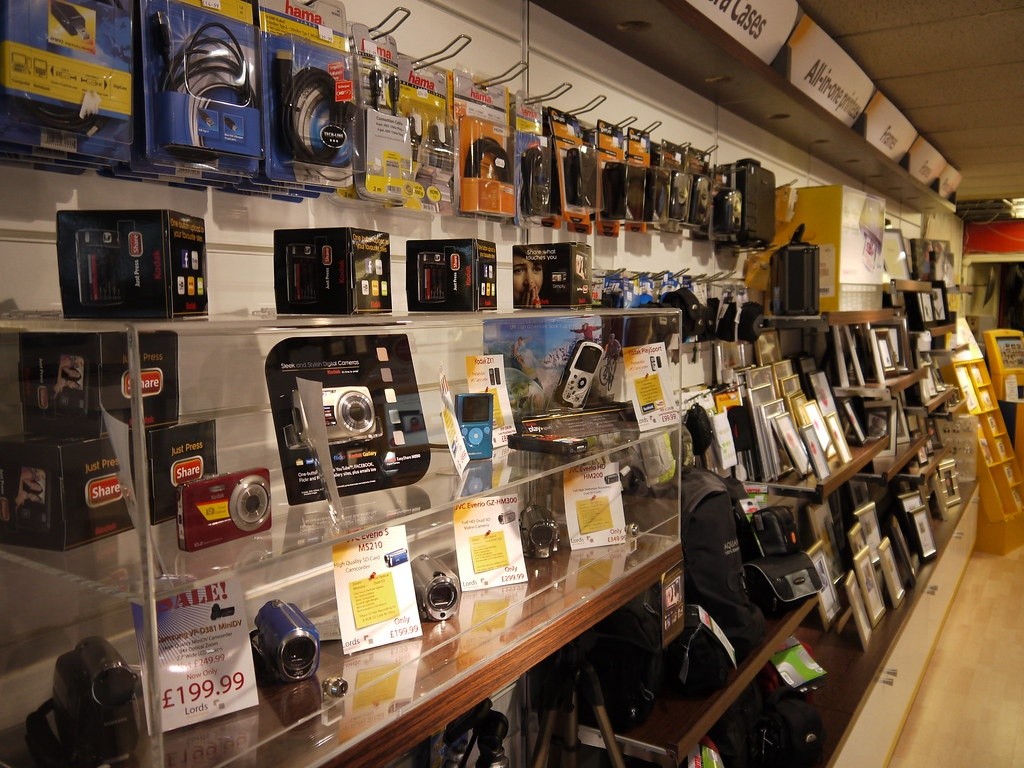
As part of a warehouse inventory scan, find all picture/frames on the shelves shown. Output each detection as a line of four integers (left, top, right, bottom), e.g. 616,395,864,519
938,458,962,507
837,570,873,652
806,539,842,632
889,515,917,589
848,522,870,557
883,228,912,280
898,491,923,523
807,498,846,585
907,504,937,563
929,474,947,521
853,501,882,567
854,545,886,628
875,537,905,609
714,281,950,481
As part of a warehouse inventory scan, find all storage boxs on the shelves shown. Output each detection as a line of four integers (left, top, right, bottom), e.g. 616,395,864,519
272,227,391,313
57,210,208,320
592,570,683,653
19,330,180,432
513,241,592,309
744,184,887,311
0,433,136,551
406,238,500,313
145,418,218,526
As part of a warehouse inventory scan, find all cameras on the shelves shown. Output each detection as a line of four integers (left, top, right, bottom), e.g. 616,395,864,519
519,504,561,558
411,555,461,622
250,599,321,683
553,341,604,409
53,635,138,768
292,386,376,448
176,466,273,552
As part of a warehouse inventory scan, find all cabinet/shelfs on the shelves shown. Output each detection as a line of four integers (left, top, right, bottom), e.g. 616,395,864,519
941,359,1024,556
580,281,969,768
0,307,683,768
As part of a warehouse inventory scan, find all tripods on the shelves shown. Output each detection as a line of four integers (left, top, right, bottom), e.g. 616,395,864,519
531,641,626,768
